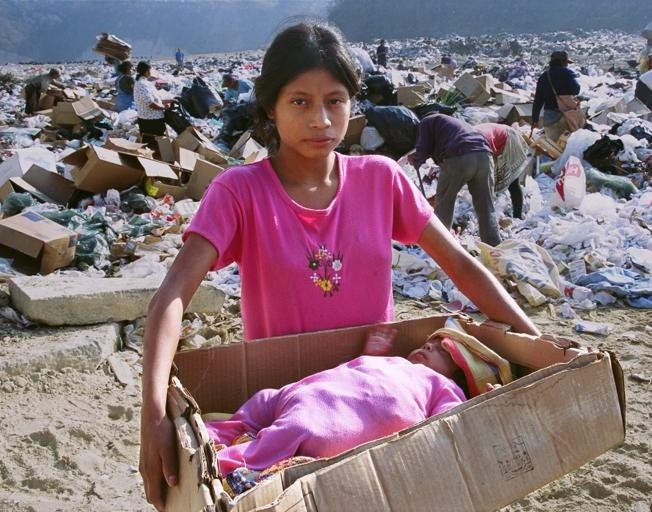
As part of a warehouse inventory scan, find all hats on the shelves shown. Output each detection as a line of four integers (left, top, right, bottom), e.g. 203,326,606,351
551,51,574,64
222,75,234,88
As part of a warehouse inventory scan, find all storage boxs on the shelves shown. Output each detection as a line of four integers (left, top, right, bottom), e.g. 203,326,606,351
395,73,494,107
498,103,544,127
165,311,627,512
175,126,226,202
0,144,144,275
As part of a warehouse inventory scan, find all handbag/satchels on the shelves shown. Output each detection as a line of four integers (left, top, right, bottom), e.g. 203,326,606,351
555,95,585,133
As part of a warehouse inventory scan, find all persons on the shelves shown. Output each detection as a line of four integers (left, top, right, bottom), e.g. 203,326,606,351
20,67,69,118
135,24,543,512
376,38,389,68
396,59,405,71
531,51,580,141
634,53,652,109
440,52,477,69
202,323,513,479
175,48,184,71
222,74,255,108
398,111,528,248
115,61,136,115
133,62,171,153
